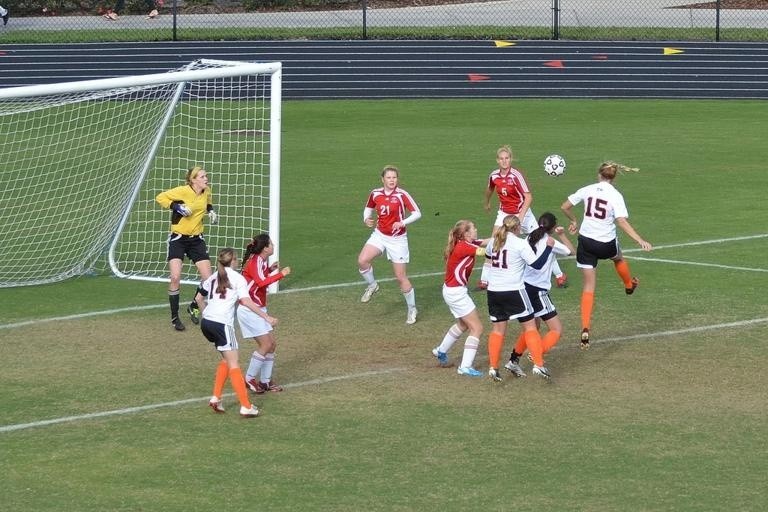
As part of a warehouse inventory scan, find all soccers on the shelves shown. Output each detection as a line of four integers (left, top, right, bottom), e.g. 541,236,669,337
542,153,568,176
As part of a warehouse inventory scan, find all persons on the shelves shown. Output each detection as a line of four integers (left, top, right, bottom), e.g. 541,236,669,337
479,145,568,289
194,247,279,417
358,166,422,324
563,161,651,351
155,166,216,330
504,213,576,378
483,215,555,381
237,233,291,394
430,220,483,376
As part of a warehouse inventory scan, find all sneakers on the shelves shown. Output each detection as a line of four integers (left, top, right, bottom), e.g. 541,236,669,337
531,365,552,380
207,395,225,415
457,366,482,376
505,360,526,378
244,374,264,394
430,347,453,368
404,307,418,326
187,304,199,324
476,280,488,291
258,379,284,393
361,282,379,304
171,318,183,332
556,272,568,289
239,405,260,416
626,276,638,295
488,368,502,383
581,329,589,352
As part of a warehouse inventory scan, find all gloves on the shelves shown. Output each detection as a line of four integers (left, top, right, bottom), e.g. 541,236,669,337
208,210,217,224
173,203,192,218
